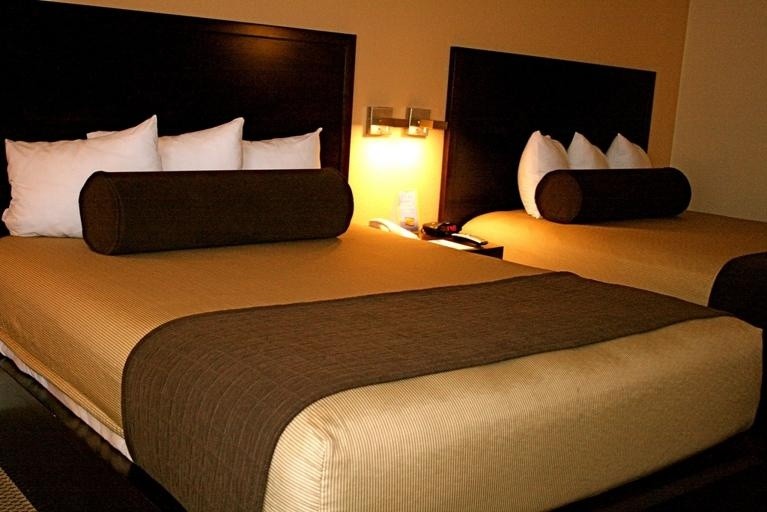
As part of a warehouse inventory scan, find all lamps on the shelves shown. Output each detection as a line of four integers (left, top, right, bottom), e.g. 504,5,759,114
362,103,409,137
405,105,447,139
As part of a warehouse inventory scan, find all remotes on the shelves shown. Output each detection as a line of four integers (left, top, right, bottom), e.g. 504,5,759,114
451,232,488,246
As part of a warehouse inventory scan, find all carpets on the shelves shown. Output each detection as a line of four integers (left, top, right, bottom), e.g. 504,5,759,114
0,467,40,512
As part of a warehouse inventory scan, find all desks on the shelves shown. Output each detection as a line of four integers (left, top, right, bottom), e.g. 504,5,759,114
417,227,504,260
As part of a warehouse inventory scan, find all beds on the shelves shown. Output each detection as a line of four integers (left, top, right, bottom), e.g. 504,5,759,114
438,44,766,317
0,225,765,511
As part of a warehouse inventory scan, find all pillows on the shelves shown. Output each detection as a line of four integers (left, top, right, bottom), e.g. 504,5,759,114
517,129,568,220
77,172,355,254
87,115,246,179
241,128,324,172
607,134,653,170
564,132,608,171
533,165,692,223
1,115,162,237
